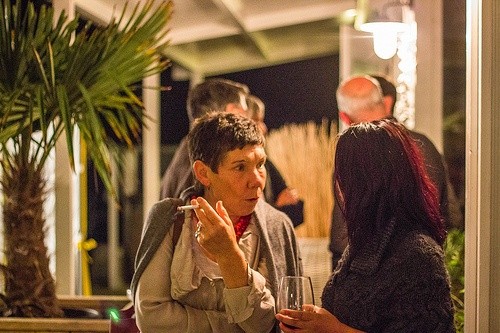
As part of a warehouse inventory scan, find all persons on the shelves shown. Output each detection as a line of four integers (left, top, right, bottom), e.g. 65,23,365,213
274,119,457,333
131,111,304,333
159,73,464,273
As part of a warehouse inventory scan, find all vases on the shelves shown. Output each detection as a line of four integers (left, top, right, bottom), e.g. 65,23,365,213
296,237,333,308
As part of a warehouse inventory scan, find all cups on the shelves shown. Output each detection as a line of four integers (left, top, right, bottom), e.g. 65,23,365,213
276,276,315,329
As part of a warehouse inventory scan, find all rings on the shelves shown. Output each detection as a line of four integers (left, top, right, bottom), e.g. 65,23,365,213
313,305,320,313
194,224,203,238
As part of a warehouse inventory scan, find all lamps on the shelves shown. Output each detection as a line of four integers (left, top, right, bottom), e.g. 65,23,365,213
354,0,403,60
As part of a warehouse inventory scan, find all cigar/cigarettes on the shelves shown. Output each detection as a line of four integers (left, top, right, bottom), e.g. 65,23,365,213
176,204,202,213
